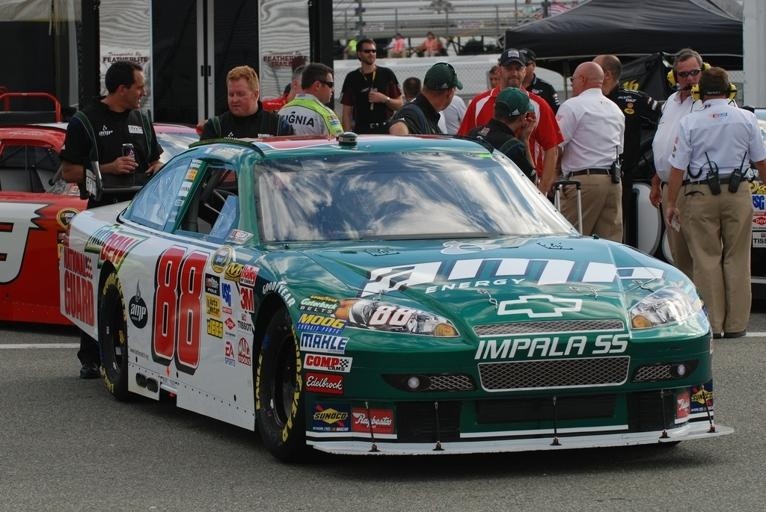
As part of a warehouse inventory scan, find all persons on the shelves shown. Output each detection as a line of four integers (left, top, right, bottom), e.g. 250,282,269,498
665,67,766,339
59,60,165,379
649,48,737,284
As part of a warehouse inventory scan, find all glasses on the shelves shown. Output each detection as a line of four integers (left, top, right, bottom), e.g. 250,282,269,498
360,49,377,53
320,80,335,88
677,66,702,78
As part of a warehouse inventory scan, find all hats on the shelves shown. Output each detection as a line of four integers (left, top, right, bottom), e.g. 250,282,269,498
424,63,463,90
495,87,534,115
501,48,537,67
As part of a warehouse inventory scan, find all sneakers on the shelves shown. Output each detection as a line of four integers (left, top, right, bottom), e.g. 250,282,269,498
80,360,100,380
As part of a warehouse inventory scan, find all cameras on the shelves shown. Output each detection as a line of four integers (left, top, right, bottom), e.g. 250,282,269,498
122,143,135,157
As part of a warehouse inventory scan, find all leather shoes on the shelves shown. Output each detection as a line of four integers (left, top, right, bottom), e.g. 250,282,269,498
713,329,746,338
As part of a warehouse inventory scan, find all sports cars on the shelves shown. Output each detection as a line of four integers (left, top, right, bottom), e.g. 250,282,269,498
0,121,202,327
67,131,735,467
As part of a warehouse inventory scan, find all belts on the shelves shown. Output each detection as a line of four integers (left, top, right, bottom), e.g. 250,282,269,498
567,168,612,177
693,177,749,184
367,121,386,129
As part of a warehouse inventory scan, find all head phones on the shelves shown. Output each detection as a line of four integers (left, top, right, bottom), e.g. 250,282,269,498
691,83,738,101
667,61,712,86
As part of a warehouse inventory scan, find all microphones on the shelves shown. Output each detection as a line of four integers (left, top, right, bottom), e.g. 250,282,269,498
667,83,692,92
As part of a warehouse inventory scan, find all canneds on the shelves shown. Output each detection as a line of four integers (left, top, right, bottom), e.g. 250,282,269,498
121,144,135,159
